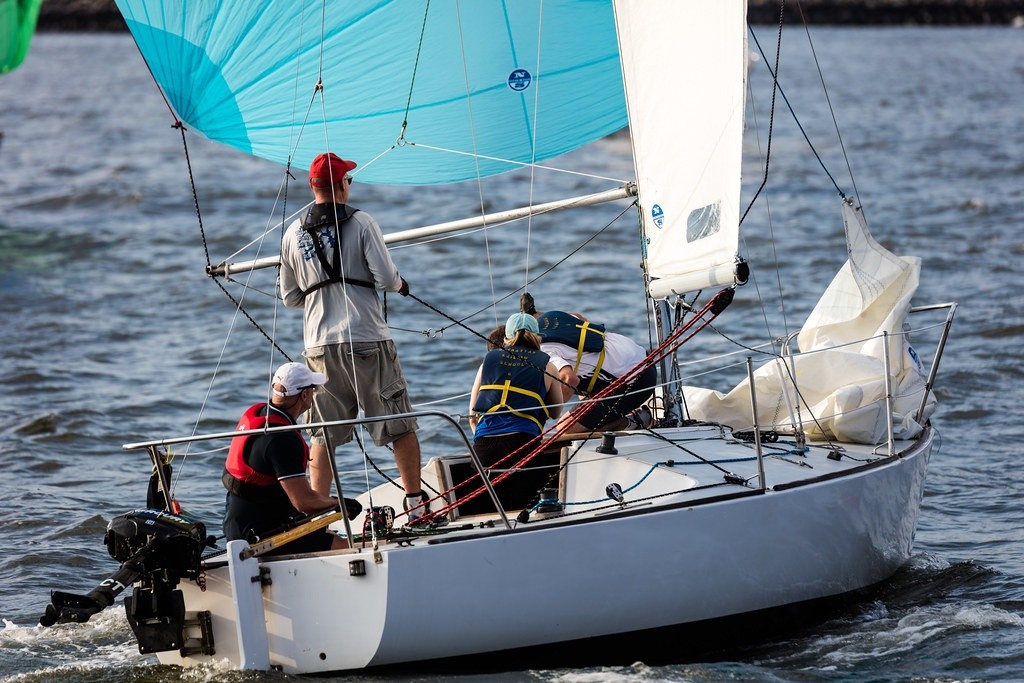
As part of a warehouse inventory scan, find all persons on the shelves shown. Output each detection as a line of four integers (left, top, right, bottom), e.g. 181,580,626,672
279,153,451,531
466,311,565,515
488,292,657,434
222,361,363,556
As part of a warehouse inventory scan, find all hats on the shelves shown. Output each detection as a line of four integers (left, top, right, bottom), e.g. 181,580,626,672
506,312,539,339
271,362,326,396
309,153,357,187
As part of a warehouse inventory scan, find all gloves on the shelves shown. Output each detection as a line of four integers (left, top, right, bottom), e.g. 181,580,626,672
398,277,409,297
332,496,363,521
520,293,537,315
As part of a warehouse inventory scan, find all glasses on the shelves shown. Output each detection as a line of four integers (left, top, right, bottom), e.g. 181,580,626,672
344,175,353,185
297,385,316,390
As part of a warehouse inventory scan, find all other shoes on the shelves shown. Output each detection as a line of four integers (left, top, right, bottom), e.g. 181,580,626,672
408,508,449,529
636,409,655,429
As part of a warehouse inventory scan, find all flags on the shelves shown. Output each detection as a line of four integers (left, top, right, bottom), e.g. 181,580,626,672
613,0,748,301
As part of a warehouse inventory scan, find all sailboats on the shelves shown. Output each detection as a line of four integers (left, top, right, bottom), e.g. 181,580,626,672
113,1,958,677
